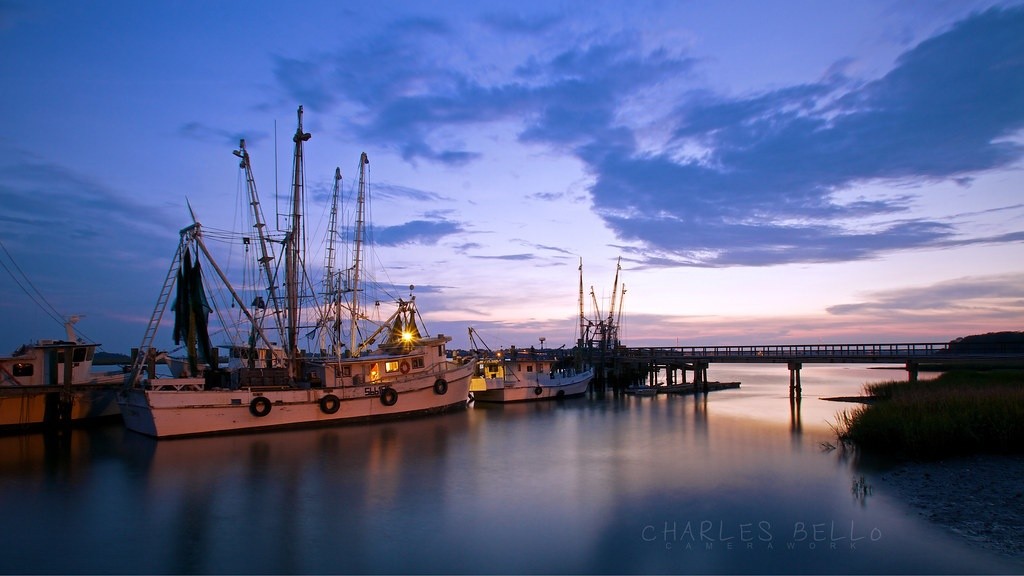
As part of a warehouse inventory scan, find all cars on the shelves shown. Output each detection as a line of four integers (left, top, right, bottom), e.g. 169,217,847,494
666,350,683,356
935,348,950,354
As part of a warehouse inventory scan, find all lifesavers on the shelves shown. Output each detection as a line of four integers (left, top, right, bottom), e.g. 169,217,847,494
556,390,565,396
534,386,543,395
318,394,340,415
434,378,449,395
249,396,273,418
380,388,398,407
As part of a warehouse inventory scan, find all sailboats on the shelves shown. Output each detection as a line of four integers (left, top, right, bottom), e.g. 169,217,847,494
119,103,473,441
552,256,649,377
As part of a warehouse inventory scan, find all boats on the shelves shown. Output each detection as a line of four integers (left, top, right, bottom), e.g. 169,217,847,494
635,389,658,395
623,384,649,394
469,348,594,404
0,312,146,426
